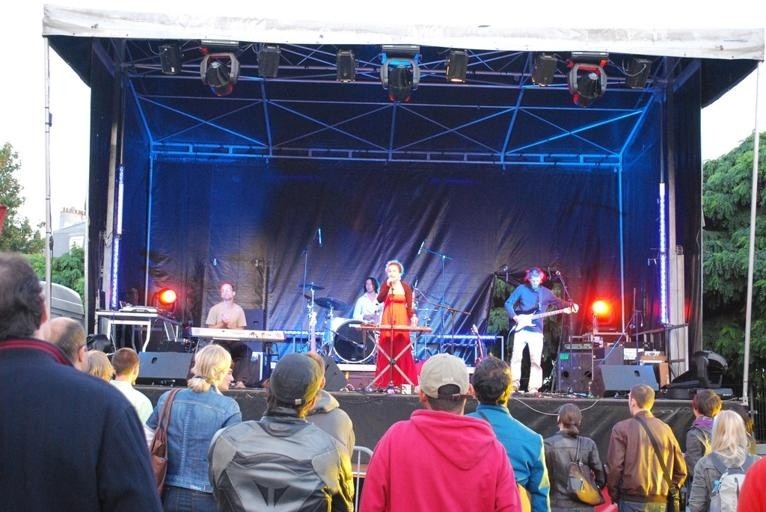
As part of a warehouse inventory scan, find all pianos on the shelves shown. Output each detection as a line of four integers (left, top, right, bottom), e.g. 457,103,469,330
349,323,432,332
191,327,285,342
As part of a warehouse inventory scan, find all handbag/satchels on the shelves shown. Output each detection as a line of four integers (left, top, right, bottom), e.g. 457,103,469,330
635,416,680,512
150,386,182,497
568,436,606,505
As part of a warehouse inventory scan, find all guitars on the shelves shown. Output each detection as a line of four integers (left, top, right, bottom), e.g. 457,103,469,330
471,324,484,366
510,304,577,333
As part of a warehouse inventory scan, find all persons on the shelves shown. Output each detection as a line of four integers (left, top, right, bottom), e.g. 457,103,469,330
352,277,384,325
207,352,354,512
542,403,605,512
687,389,762,512
144,344,242,512
205,281,252,389
504,267,573,393
374,261,418,391
605,385,688,512
358,353,551,512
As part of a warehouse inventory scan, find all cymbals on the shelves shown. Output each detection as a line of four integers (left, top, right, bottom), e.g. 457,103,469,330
299,283,325,290
315,297,347,311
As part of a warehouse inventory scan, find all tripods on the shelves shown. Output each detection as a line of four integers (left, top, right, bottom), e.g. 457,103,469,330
318,306,346,364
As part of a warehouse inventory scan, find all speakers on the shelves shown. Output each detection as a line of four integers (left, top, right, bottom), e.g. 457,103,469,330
557,352,593,392
136,351,192,380
594,362,660,397
322,355,349,392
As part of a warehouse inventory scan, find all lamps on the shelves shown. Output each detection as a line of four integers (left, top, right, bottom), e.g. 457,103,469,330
195,47,239,98
158,43,184,77
568,52,610,111
621,56,659,92
382,46,421,104
530,54,560,88
445,49,473,83
258,43,280,81
338,49,357,85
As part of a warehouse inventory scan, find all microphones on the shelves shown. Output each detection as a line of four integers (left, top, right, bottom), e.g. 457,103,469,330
317,228,323,247
389,281,395,292
412,279,418,298
417,241,426,256
556,271,565,286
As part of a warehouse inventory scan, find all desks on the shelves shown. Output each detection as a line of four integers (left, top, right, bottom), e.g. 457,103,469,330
94,311,185,353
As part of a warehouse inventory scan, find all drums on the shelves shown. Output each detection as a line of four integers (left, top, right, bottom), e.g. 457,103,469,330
333,319,378,364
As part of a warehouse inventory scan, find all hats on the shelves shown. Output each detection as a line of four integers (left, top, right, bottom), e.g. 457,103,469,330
271,353,321,405
417,353,473,401
